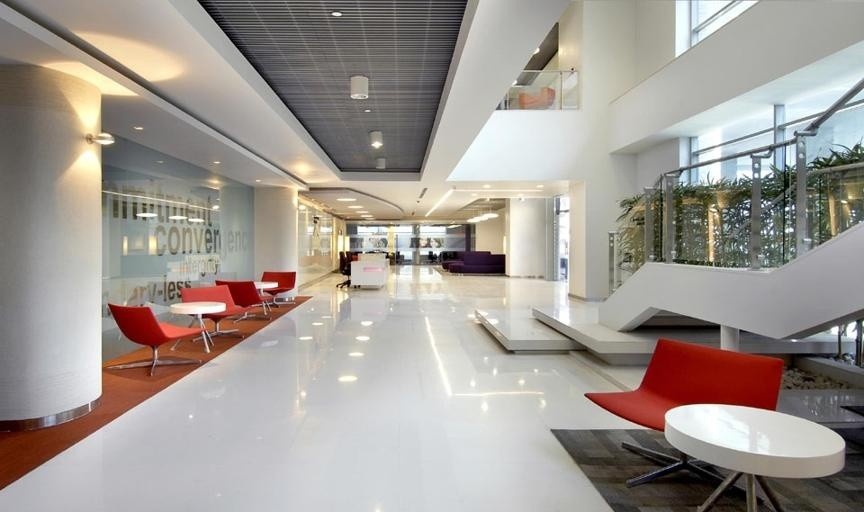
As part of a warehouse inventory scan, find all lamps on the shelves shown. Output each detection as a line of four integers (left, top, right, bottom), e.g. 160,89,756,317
87,132,115,146
371,132,383,148
350,76,368,100
376,158,387,168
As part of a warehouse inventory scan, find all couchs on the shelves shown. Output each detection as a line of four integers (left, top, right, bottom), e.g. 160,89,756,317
441,251,505,273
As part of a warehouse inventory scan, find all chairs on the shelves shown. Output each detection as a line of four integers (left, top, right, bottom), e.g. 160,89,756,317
428,251,438,264
180,284,248,342
252,272,297,309
519,88,556,109
215,280,274,324
336,251,360,288
102,303,209,377
397,255,404,264
583,338,786,490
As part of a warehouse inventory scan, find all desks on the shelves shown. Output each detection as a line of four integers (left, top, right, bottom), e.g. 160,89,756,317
663,402,847,511
253,281,278,315
169,300,227,354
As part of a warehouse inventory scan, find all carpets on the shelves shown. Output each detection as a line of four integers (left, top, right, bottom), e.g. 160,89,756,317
551,426,863,511
0,296,314,487
